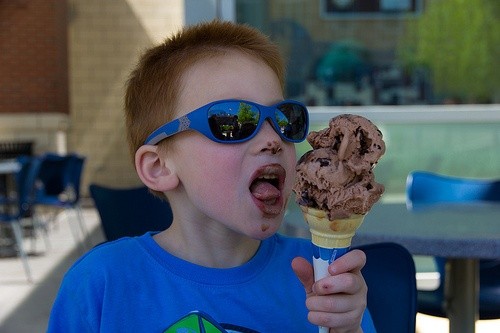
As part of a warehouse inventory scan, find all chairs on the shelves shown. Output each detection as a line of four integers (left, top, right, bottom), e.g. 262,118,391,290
0,152,88,279
89,185,173,242
406,172,500,318
348,241,417,333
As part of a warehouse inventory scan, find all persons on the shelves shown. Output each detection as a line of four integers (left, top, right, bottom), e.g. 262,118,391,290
45,20,379,333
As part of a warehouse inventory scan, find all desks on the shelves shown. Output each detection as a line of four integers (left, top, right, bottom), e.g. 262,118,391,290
281,190,499,333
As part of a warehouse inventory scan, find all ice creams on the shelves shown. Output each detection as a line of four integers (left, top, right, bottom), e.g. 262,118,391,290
290,114,387,333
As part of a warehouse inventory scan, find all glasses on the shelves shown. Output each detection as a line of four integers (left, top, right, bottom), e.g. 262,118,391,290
144,97,310,145
